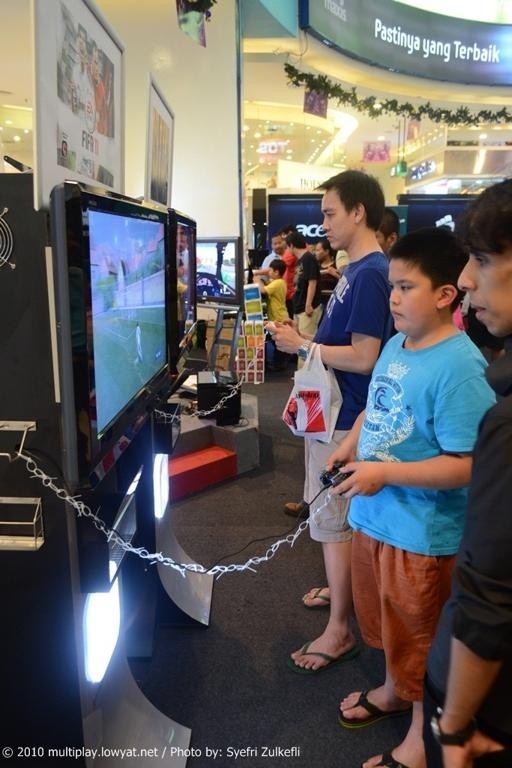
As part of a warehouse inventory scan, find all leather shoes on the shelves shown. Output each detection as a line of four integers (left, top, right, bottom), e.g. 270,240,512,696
284,499,308,516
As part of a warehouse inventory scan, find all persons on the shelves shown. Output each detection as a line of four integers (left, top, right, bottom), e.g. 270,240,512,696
135,322,144,363
67,24,113,138
176,226,191,319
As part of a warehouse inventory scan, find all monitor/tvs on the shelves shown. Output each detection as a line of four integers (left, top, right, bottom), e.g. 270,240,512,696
50,178,173,491
268,194,326,256
397,193,480,239
199,236,243,304
169,207,197,365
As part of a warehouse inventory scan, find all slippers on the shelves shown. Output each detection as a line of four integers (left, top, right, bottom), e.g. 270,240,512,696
337,682,413,730
361,741,429,768
302,585,332,607
287,633,364,675
